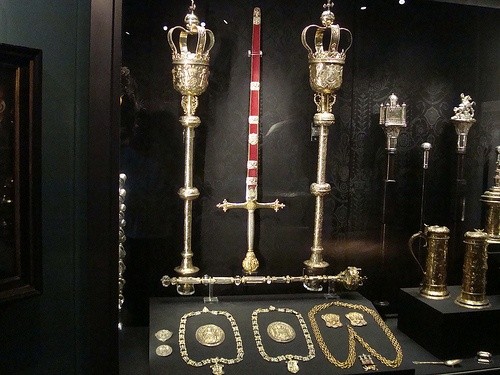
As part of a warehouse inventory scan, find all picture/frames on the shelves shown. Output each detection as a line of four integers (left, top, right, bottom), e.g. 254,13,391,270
0,42,43,304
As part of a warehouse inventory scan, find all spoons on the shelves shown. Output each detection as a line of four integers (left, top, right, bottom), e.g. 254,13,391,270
412,358,463,366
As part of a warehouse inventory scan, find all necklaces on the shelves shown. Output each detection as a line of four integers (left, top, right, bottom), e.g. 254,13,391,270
178,306,245,375
307,301,403,369
252,305,316,374
